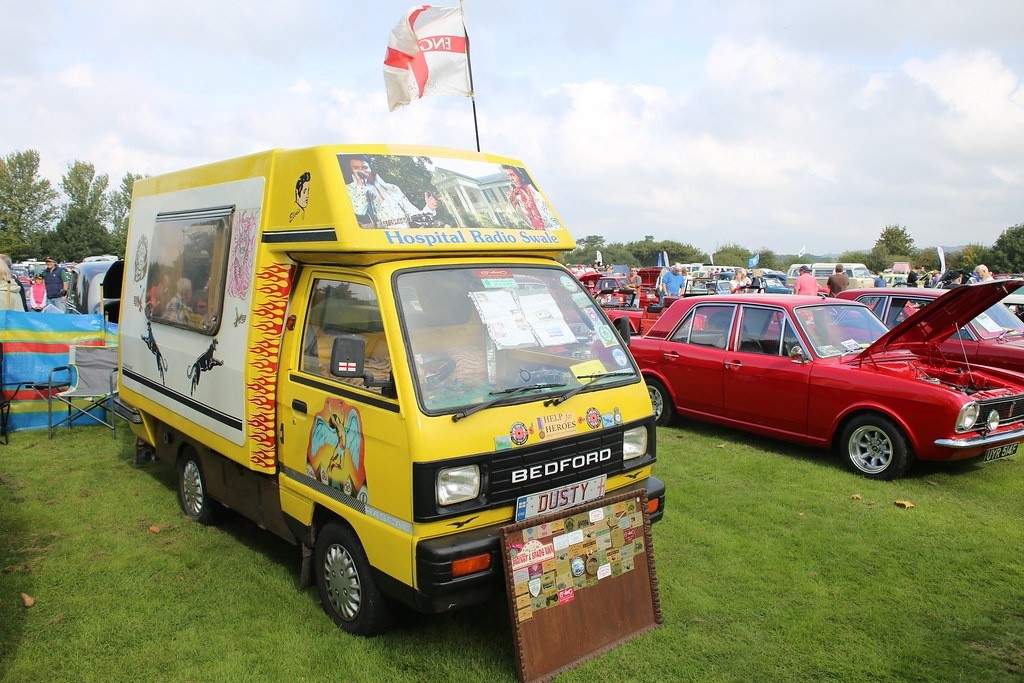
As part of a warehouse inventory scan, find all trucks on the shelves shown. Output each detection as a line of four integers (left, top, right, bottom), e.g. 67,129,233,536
115,143,669,640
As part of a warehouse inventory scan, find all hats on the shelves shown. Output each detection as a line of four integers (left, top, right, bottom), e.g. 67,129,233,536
46,257,55,261
34,273,44,280
798,266,809,272
578,264,583,269
951,272,960,279
566,263,571,267
836,264,844,271
676,263,681,271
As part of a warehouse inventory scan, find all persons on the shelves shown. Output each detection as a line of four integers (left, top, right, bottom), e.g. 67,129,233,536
566,263,601,275
943,272,962,289
925,270,940,285
975,264,993,282
148,273,208,323
625,264,643,308
907,264,921,287
345,154,438,228
660,263,688,306
502,164,562,231
30,255,67,313
708,268,725,280
874,275,886,287
795,266,818,296
0,254,29,312
730,269,765,294
827,263,849,297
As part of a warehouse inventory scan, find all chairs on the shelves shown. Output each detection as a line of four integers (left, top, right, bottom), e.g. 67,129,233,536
724,319,764,354
47,344,120,439
779,318,803,356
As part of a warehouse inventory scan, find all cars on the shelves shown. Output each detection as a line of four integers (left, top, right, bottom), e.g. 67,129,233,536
9,255,119,317
802,287,1024,377
565,262,1024,340
623,278,1024,482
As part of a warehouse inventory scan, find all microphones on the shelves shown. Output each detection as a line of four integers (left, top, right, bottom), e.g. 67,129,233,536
357,172,365,178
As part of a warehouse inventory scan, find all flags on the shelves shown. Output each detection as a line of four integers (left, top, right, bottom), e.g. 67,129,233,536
657,250,670,267
748,254,759,267
799,247,805,258
596,250,602,265
383,4,471,113
937,245,946,276
